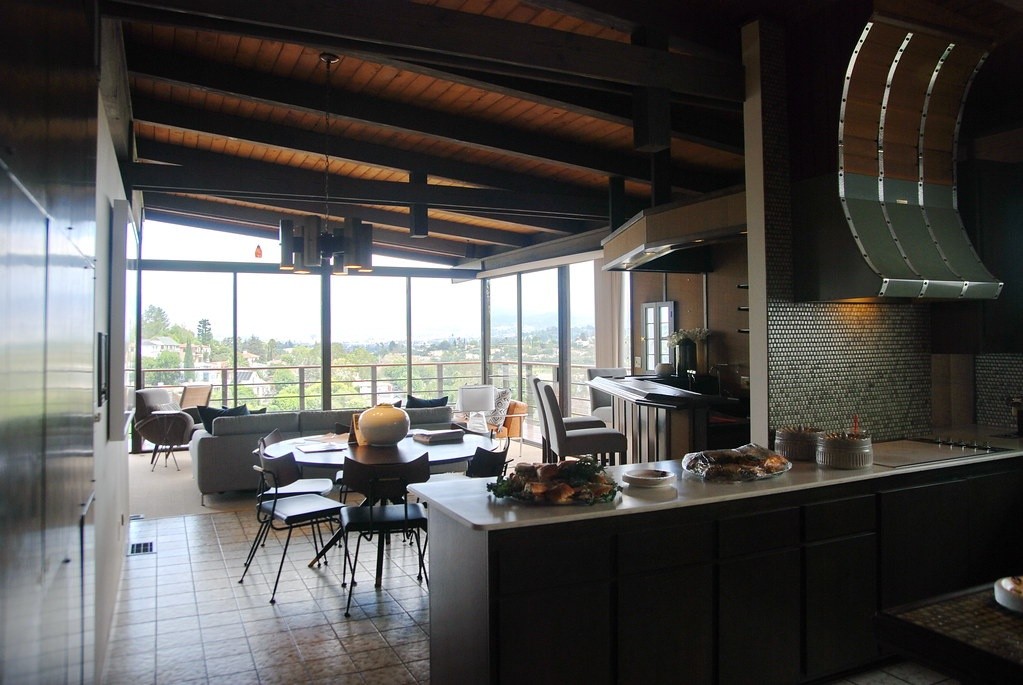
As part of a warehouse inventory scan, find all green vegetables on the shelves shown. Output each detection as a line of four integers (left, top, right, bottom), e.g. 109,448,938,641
485,475,535,503
554,459,619,505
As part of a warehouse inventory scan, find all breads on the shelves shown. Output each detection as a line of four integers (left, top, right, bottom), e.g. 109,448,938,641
686,442,789,483
507,459,612,504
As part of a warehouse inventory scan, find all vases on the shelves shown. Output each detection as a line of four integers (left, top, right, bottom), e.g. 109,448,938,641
675,344,695,376
695,335,708,376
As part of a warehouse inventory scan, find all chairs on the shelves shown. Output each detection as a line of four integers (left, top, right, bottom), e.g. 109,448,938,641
529,377,607,466
238,423,514,617
151,383,214,465
537,382,628,465
587,368,628,429
135,388,202,472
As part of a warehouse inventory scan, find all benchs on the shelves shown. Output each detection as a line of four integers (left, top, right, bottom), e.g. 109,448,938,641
452,399,528,457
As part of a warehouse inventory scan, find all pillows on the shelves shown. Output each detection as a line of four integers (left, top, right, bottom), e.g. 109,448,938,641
222,406,266,414
152,401,182,412
406,394,448,408
466,387,512,426
197,405,250,435
368,400,402,409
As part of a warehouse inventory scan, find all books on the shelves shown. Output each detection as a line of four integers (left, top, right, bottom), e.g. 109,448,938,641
413,429,465,443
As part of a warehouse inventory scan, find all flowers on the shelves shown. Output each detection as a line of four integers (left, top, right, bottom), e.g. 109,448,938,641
668,328,711,349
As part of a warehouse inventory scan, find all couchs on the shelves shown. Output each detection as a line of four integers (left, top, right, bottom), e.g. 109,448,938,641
189,407,467,507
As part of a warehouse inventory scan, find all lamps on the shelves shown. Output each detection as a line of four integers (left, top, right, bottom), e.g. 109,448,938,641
278,51,373,274
456,385,495,434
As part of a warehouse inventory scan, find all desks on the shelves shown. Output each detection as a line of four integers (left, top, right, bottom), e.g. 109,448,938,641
264,429,500,590
879,579,1023,685
586,374,709,464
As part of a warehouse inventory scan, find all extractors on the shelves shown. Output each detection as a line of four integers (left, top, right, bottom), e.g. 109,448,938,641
794,20,1003,305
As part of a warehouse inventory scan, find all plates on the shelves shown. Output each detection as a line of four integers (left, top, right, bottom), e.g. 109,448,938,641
682,455,792,478
622,470,677,487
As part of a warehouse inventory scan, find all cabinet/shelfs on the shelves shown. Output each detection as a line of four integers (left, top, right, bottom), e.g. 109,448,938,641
427,457,1023,685
